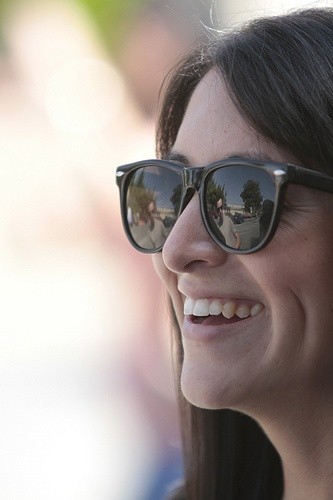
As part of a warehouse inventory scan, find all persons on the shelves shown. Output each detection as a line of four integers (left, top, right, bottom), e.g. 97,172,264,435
115,8,333,500
259,200,274,241
216,197,241,249
145,200,170,248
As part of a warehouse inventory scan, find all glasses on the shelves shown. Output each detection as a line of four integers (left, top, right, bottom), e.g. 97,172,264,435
116,158,333,254
